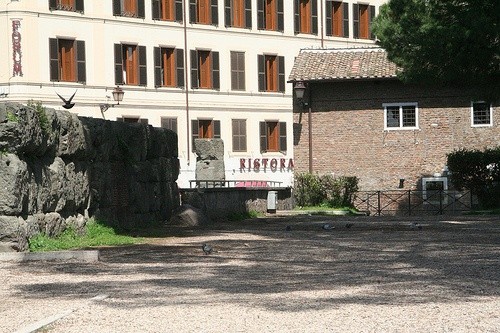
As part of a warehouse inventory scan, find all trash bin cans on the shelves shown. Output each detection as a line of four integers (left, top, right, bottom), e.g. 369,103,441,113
266,191,278,214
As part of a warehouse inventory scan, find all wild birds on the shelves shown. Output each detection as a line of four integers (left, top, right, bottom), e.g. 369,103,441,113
346,223,355,228
55,89,78,109
202,243,213,255
321,223,337,230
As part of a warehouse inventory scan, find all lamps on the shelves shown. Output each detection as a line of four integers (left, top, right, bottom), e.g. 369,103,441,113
293,79,311,111
100,85,126,113
